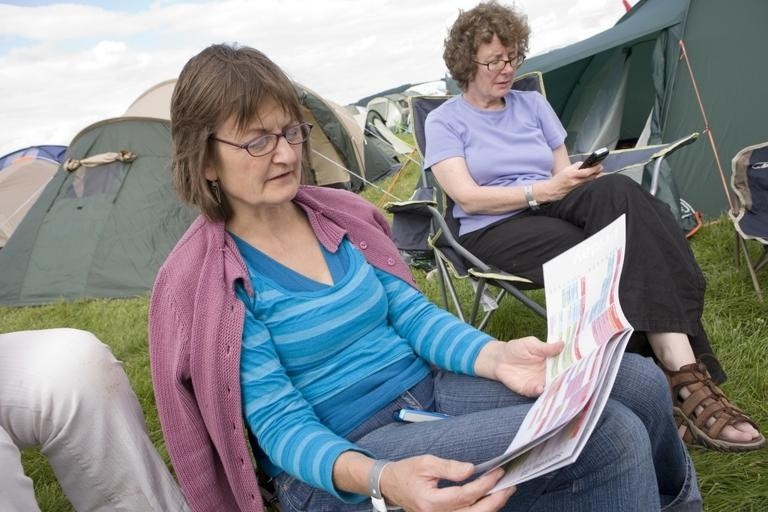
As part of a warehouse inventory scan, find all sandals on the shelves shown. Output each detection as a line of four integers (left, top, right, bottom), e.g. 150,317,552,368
667,354,765,453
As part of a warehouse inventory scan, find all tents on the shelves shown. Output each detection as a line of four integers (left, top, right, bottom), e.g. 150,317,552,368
1,1,767,308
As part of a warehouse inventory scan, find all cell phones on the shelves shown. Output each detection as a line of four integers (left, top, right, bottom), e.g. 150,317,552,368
578,147,609,169
392,408,448,422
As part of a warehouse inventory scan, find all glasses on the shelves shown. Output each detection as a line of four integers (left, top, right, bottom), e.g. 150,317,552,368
472,52,525,71
210,121,313,157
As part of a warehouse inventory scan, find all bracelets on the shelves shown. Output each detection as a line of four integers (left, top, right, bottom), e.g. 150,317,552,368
367,458,401,512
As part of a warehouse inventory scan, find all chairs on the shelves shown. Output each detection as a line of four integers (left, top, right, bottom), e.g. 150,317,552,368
724,142,767,305
379,69,702,333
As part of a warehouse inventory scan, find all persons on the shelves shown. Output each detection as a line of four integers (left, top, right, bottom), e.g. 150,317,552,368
0,327,191,512
149,41,704,511
425,2,766,453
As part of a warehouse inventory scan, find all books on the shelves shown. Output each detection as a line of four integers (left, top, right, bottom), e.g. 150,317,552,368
475,211,638,496
